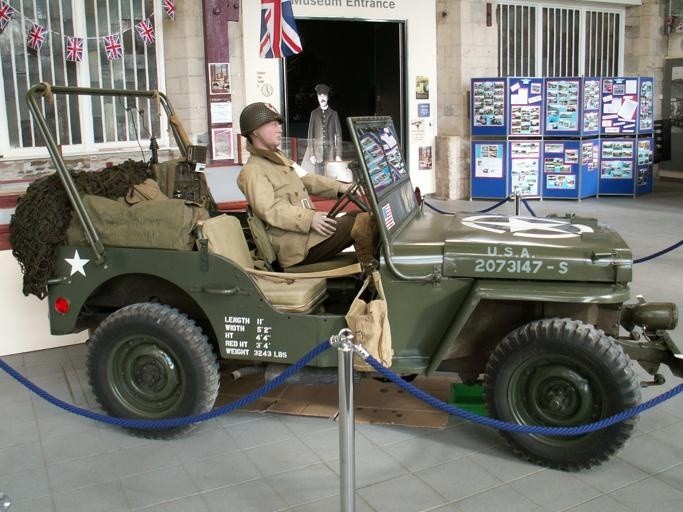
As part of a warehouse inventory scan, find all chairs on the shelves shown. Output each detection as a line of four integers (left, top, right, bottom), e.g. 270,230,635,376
195,206,358,322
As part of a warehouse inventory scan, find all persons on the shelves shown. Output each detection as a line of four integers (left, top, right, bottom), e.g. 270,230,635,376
308,86,343,178
237,101,381,301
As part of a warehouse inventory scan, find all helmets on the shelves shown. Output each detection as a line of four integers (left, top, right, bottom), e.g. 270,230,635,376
239,102,285,135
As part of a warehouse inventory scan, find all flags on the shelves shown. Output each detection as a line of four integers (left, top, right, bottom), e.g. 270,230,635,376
0,0,176,63
259,0,302,59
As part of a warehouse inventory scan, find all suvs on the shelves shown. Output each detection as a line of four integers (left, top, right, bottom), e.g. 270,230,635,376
25,82,683,472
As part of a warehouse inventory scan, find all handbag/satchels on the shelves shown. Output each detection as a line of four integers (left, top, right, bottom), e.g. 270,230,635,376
323,155,355,184
343,274,392,373
